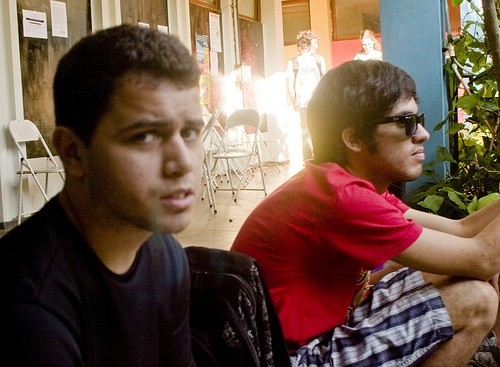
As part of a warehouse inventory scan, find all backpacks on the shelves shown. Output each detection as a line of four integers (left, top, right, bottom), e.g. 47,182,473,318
184,246,294,367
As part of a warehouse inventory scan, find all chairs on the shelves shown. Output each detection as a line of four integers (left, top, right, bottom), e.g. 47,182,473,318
201,108,282,223
7,119,66,226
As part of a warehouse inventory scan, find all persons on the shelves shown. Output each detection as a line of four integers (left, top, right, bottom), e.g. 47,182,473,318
231,30,500,367
0,24,205,367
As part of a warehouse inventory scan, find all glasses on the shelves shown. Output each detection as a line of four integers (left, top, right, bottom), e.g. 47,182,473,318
367,113,424,137
